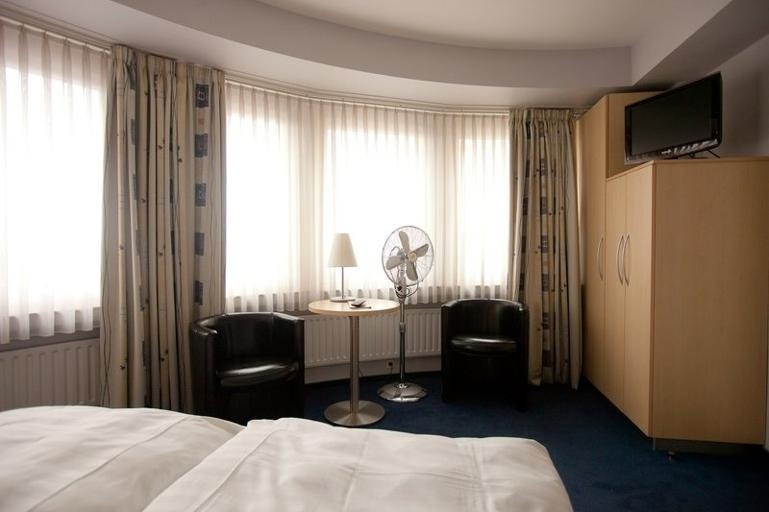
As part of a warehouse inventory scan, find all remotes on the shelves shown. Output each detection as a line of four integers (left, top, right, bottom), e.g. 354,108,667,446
350,298,367,307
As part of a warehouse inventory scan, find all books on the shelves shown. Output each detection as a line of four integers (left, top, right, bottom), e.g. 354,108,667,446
346,300,372,310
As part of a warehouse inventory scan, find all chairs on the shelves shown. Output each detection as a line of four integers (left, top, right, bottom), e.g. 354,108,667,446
189,311,303,427
439,298,529,403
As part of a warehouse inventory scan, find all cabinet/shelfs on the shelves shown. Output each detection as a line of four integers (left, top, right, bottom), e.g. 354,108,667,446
569,90,667,386
601,155,769,455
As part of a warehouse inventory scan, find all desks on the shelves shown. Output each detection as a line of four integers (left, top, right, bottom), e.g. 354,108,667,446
307,298,401,427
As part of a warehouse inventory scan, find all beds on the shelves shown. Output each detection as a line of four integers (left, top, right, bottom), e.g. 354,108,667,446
140,417,572,510
2,403,243,510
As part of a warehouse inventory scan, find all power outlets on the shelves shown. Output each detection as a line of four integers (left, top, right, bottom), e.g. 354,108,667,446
386,359,394,368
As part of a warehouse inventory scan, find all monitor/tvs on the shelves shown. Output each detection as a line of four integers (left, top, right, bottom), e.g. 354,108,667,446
623,69,724,163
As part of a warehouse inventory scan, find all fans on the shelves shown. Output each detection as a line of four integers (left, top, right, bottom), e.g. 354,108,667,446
378,225,434,403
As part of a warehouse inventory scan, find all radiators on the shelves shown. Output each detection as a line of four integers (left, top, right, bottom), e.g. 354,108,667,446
299,307,443,369
0,338,102,412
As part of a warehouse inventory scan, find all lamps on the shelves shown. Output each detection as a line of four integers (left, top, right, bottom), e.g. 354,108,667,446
329,231,358,302
